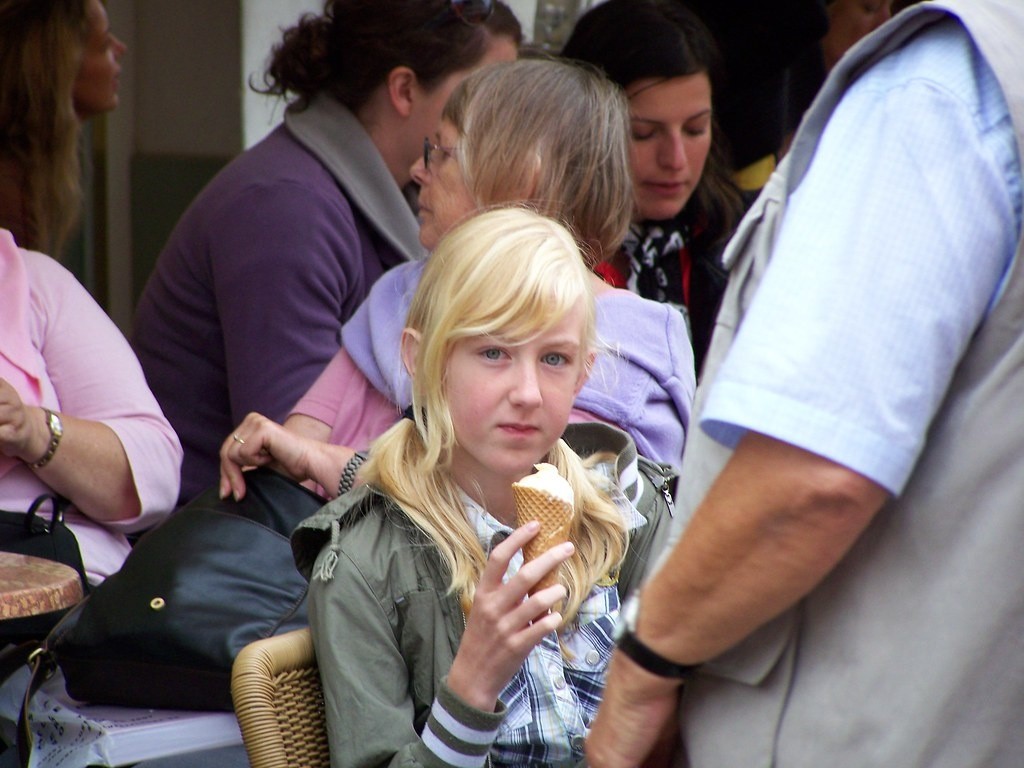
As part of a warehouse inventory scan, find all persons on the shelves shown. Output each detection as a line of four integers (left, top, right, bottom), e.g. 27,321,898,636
0,0,1024,768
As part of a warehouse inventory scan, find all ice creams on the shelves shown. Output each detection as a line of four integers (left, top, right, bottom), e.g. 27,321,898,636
510,462,574,647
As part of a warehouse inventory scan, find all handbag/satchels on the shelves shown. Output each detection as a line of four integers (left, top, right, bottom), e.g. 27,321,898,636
17,467,325,768
0,495,93,638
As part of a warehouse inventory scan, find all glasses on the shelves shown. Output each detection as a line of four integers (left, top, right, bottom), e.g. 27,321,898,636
423,134,461,168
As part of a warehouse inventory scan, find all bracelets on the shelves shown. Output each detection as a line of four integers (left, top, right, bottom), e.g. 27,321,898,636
616,626,706,679
338,451,368,497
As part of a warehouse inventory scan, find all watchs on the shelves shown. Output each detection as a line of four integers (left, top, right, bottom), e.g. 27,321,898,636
27,407,62,469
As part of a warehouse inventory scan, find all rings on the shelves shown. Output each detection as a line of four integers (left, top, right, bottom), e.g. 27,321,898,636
233,436,245,445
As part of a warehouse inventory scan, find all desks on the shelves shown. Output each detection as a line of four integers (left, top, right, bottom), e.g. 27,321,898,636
1,661,250,768
